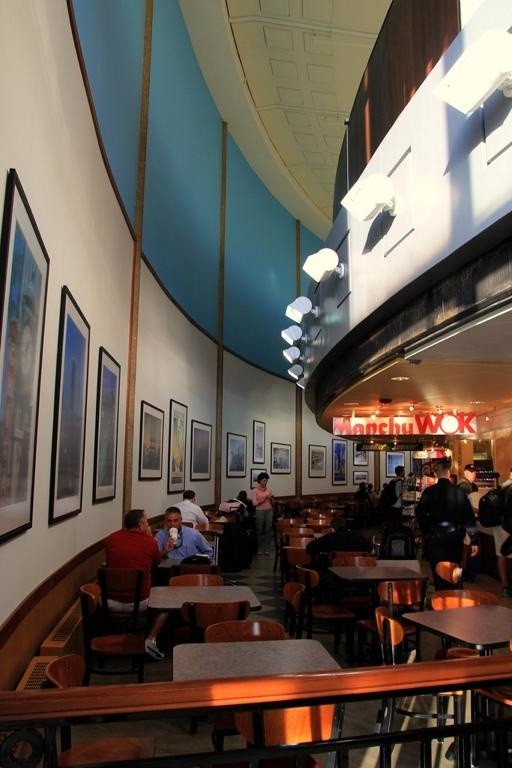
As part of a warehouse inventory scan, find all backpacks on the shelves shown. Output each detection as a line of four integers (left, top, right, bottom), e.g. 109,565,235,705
384,480,399,507
478,485,512,528
379,514,416,560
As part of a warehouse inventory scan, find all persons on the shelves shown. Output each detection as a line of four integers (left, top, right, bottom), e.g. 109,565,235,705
251,473,275,555
153,506,213,560
357,482,365,492
414,458,479,649
479,467,512,597
306,518,375,605
171,490,210,524
384,466,405,530
367,484,378,508
455,464,479,583
339,508,362,529
407,472,415,491
238,491,256,514
105,509,168,660
355,486,372,529
284,497,304,519
378,483,388,498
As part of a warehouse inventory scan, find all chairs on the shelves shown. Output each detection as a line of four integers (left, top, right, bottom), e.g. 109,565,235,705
44,653,157,768
434,561,464,590
284,528,315,536
235,692,341,768
78,584,146,689
281,546,312,582
182,522,193,528
206,496,380,528
357,581,422,663
183,601,250,641
296,564,355,655
278,581,305,638
373,605,434,738
429,589,497,609
331,552,377,567
196,523,223,539
204,619,287,645
170,574,224,588
98,566,149,637
286,534,315,548
178,565,214,575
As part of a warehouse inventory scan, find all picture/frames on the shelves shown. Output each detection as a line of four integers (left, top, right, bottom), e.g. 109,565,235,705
270,442,292,475
48,284,91,529
226,432,247,478
138,399,165,480
332,439,348,486
308,444,326,478
252,420,266,464
353,443,369,467
251,468,267,490
92,346,121,503
352,471,370,485
386,452,405,478
0,169,50,546
167,399,188,494
190,418,213,480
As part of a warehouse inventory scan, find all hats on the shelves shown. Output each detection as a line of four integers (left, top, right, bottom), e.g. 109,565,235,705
464,463,479,472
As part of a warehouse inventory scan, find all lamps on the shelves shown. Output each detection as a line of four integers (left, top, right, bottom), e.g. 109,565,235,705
295,376,306,389
302,248,345,287
285,295,318,323
338,167,401,224
410,402,416,413
282,346,304,364
436,23,512,118
281,325,307,345
288,364,305,380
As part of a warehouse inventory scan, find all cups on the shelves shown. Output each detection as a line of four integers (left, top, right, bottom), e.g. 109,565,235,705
168,526,178,545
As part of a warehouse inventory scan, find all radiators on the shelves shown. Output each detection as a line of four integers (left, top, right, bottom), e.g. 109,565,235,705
2,656,63,768
40,586,86,672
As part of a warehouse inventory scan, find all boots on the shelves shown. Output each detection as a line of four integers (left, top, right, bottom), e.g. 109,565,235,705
265,544,271,554
257,543,262,554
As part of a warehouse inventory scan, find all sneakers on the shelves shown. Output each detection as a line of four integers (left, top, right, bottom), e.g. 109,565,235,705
144,639,165,660
